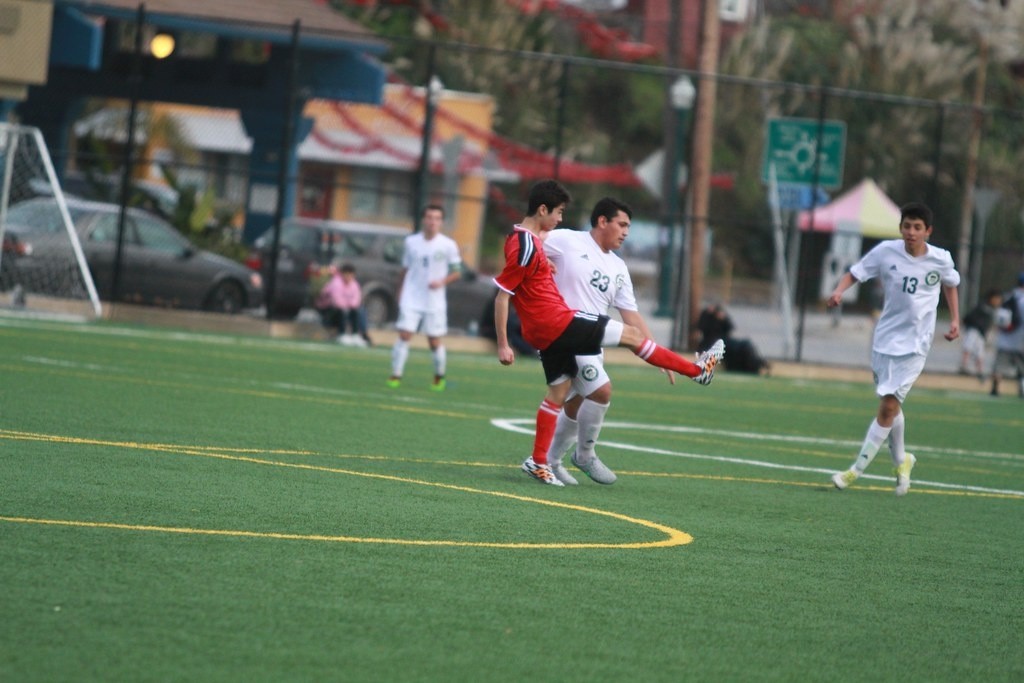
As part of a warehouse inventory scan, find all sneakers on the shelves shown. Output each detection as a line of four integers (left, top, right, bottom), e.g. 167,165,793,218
387,376,399,386
692,339,726,385
551,461,578,485
521,456,564,486
430,376,446,391
832,469,859,489
571,451,616,485
894,452,916,494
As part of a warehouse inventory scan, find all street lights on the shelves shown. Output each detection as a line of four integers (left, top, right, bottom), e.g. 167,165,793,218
652,76,695,319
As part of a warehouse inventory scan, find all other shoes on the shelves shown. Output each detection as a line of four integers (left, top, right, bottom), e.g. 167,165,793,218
339,335,368,347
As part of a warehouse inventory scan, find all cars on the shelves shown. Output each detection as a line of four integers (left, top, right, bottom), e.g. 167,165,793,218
61,172,179,220
0,198,264,314
243,217,497,326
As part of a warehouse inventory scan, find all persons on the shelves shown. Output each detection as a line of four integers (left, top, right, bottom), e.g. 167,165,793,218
316,263,369,349
385,205,464,392
698,304,772,377
538,196,675,484
492,180,725,487
990,271,1024,398
962,290,1003,377
826,202,960,497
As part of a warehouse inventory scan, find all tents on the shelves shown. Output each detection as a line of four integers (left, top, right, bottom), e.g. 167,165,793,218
797,176,929,314
